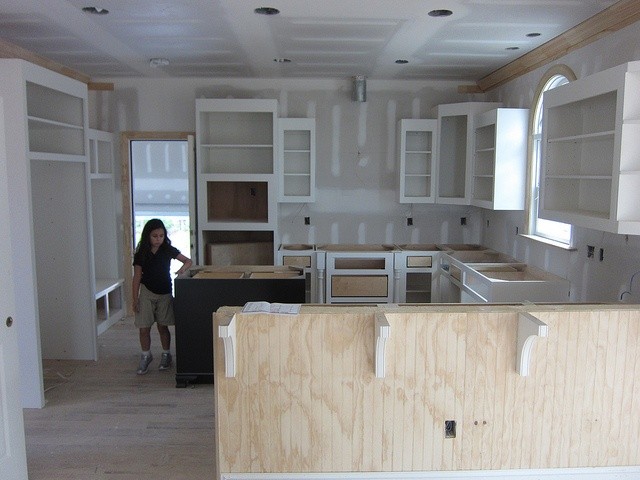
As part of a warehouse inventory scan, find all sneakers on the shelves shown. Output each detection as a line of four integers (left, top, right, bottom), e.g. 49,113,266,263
159,352,172,369
137,352,153,375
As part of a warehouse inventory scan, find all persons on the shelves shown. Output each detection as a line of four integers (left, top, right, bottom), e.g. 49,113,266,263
132,219,193,374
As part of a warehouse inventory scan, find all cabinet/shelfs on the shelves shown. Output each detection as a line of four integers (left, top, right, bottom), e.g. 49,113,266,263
88,128,128,337
397,118,436,204
0,57,100,410
472,108,530,211
174,268,306,389
278,118,316,205
394,251,441,303
432,101,504,206
195,98,278,268
441,251,570,303
278,243,315,304
538,60,640,236
326,252,394,304
